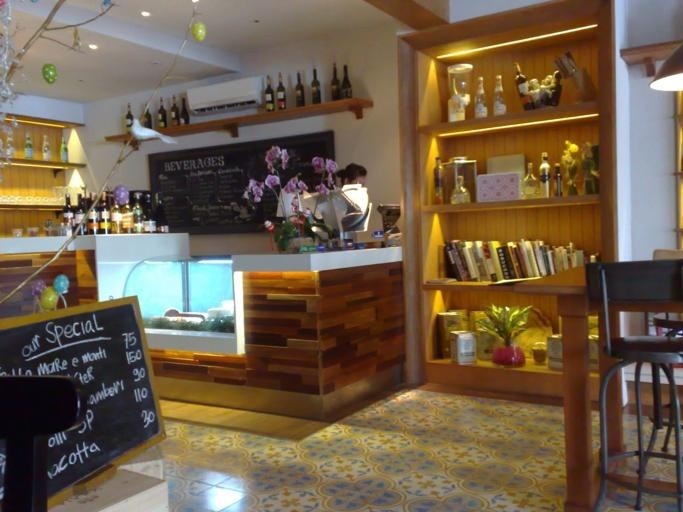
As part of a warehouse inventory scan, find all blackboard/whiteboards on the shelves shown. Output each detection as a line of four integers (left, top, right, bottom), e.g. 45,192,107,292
0,296,167,511
148,130,336,234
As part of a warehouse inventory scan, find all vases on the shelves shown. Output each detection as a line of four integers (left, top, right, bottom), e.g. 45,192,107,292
273,236,313,254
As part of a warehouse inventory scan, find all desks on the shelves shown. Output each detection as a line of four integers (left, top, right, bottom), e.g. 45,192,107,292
513,265,683,512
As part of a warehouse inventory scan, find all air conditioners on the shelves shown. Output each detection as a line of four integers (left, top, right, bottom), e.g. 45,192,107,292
187,75,265,116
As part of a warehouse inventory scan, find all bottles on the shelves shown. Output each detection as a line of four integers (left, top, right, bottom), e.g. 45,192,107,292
474,74,507,118
42,135,51,160
523,151,562,199
450,176,471,205
125,94,189,134
25,134,33,158
433,157,444,206
551,70,561,106
62,190,169,235
60,136,68,162
264,63,352,112
511,61,535,111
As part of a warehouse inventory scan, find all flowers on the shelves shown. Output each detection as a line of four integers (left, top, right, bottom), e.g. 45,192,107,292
244,146,340,250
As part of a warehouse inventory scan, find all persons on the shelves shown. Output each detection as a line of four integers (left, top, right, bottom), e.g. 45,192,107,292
335,163,368,188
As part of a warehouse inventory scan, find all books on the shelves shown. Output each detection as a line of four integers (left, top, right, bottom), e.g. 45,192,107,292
442,239,597,281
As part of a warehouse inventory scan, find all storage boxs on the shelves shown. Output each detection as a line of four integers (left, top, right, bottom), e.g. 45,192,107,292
546,333,599,371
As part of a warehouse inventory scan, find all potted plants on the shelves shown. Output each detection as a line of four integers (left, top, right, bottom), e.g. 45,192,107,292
473,304,533,367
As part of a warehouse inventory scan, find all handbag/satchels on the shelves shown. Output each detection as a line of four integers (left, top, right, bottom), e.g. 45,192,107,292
301,183,372,232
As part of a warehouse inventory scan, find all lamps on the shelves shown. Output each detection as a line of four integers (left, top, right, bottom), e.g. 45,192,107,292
645,45,683,94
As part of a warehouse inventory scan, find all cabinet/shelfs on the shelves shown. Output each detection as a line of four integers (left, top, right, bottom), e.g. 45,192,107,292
395,0,617,402
0,112,86,211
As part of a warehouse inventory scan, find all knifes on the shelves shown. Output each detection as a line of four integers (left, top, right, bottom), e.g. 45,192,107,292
554,53,578,76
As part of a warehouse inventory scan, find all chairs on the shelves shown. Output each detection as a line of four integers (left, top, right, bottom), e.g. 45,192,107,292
634,248,683,475
584,260,683,512
